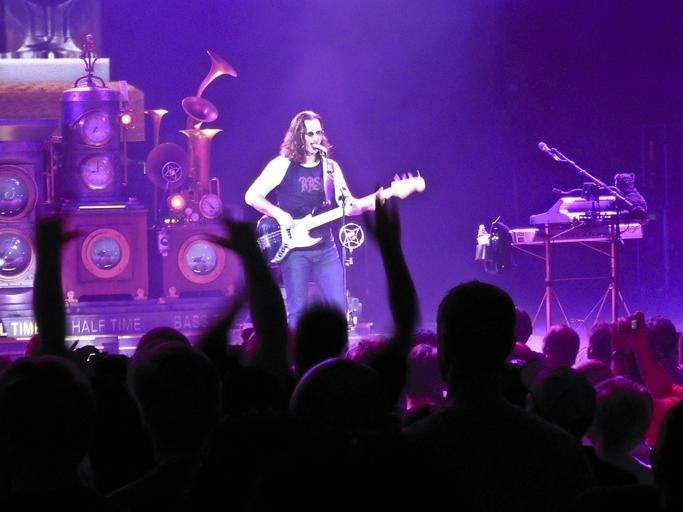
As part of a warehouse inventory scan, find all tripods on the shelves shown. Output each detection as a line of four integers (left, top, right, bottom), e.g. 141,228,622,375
593,247,634,326
532,235,570,333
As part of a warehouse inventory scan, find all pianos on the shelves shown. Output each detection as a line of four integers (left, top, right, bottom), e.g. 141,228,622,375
477,197,643,247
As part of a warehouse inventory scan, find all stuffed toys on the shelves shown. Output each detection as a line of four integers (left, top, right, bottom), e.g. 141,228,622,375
606,173,647,217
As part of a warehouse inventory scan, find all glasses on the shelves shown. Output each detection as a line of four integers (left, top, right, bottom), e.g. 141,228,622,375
299,129,325,139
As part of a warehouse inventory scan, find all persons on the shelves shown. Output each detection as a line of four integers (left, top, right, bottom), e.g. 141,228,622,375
1,192,683,512
244,111,384,329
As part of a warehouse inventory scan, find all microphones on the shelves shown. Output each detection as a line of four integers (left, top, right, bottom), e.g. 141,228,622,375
537,142,561,162
311,143,328,154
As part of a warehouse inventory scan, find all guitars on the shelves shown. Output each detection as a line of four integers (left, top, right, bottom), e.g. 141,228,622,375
255,169,426,263
